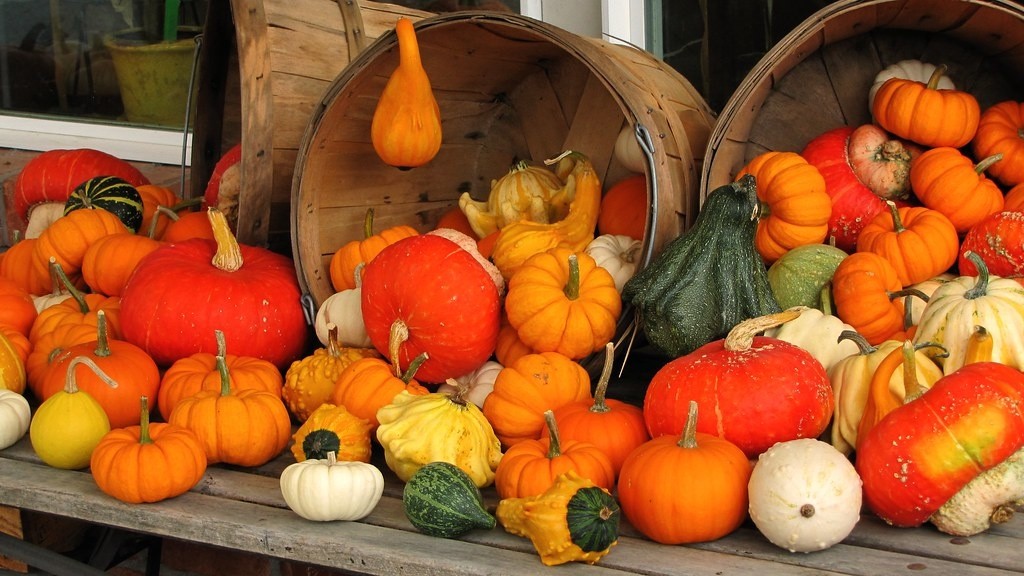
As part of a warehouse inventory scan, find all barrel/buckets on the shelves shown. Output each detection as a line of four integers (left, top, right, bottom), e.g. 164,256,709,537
698,0,1024,216
181,1,438,253
104,25,205,128
291,12,718,369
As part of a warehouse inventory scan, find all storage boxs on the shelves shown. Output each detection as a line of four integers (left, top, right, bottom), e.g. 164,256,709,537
0,506,89,573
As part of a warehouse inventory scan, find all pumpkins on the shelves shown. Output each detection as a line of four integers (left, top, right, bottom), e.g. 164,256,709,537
0,16,1024,563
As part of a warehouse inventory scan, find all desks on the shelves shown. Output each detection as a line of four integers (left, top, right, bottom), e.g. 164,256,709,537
1,403,1023,576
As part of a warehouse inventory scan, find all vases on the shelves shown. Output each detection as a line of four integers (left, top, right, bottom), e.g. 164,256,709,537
103,25,203,128
